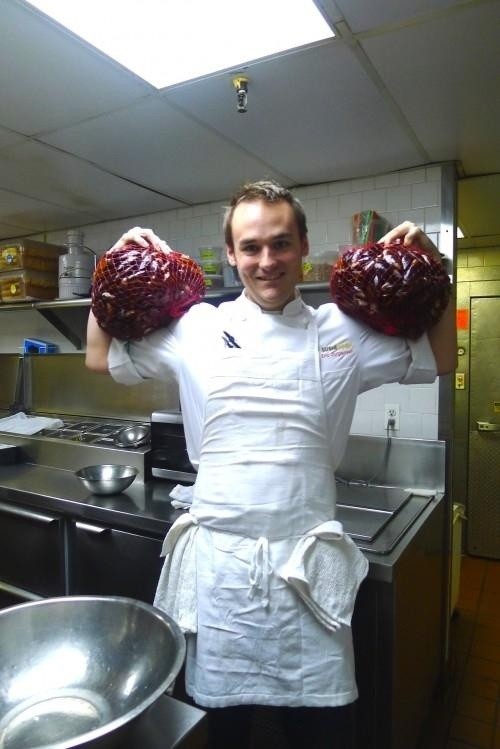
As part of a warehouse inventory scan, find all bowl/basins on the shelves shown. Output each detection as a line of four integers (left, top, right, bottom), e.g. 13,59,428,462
74,463,138,496
112,420,152,447
0,594,187,749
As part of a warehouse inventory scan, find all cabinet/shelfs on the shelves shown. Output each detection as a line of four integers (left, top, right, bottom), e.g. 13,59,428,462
0,500,63,609
63,514,170,609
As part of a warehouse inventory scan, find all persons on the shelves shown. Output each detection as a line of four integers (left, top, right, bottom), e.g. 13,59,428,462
84,179,459,748
